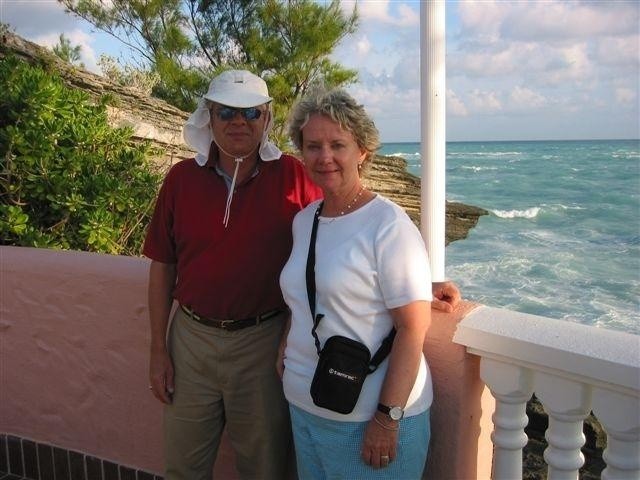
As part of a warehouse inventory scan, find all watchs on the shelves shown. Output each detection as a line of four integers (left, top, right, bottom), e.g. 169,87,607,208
375,402,405,422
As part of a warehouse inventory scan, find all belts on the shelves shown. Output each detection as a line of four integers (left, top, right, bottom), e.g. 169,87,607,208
180,306,284,330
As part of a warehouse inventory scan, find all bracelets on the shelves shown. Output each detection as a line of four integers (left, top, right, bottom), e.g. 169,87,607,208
371,415,400,433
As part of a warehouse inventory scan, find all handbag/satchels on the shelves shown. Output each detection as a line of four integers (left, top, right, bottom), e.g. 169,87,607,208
310,336,370,414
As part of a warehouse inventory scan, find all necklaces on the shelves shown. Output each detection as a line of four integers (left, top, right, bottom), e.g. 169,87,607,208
314,185,368,225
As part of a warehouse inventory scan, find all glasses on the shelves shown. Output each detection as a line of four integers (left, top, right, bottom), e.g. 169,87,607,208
210,106,266,121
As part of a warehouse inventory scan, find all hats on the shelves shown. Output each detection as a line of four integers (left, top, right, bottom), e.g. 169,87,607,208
204,68,273,108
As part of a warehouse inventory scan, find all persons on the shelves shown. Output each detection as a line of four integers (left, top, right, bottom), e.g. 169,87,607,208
140,66,464,480
272,86,438,480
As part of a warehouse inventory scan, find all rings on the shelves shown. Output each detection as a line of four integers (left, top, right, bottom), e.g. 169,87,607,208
380,455,390,459
147,384,155,391
